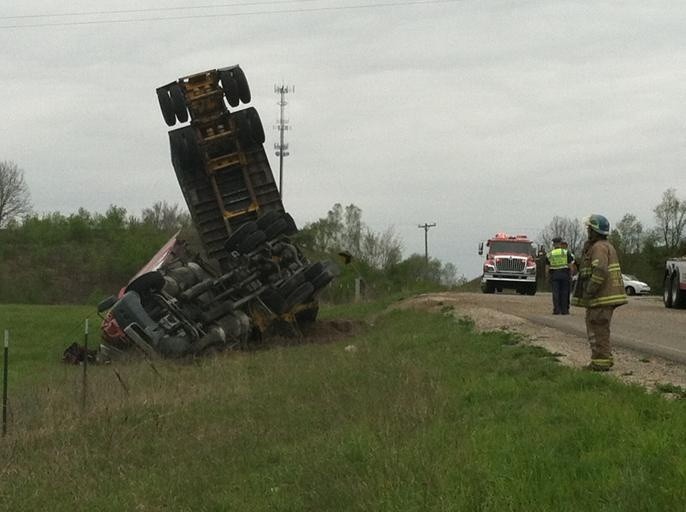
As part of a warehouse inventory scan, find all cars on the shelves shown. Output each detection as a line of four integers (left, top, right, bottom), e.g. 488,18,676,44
570,271,650,296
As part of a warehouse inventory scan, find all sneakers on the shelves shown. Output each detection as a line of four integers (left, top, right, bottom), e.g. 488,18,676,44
582,361,614,371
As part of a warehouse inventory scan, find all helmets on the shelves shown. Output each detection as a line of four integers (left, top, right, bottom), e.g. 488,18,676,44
583,214,611,236
561,240,568,248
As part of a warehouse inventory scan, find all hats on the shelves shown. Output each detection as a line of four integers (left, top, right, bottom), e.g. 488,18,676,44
552,236,562,241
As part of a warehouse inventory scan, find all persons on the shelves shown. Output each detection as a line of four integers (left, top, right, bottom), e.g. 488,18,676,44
571,214,630,372
560,242,577,310
545,236,578,314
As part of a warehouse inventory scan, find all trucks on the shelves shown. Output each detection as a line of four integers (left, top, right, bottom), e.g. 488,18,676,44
662,257,686,308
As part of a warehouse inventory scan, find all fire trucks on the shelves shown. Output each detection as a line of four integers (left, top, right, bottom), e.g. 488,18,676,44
478,234,537,295
